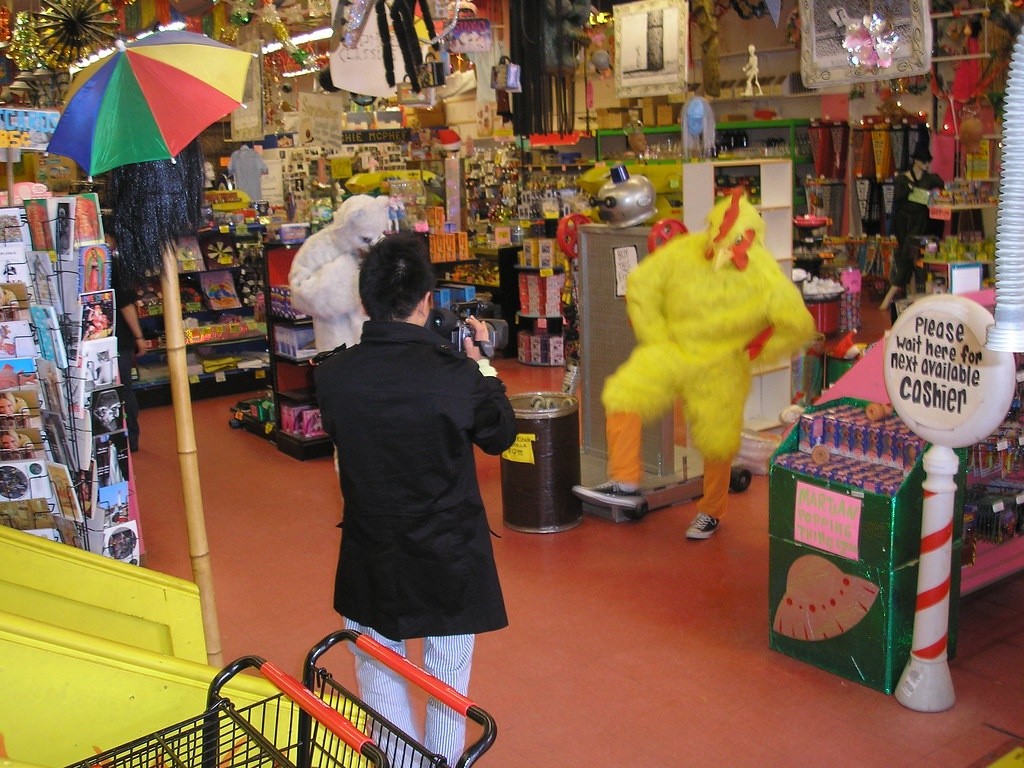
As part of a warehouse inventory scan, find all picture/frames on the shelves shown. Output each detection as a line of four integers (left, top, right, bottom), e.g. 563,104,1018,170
798,0,935,88
612,0,689,100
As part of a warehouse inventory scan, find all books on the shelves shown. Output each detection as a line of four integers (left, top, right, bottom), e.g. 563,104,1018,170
0,190,147,569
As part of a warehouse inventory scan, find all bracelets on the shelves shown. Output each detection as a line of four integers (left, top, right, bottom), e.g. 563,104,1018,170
135,334,145,340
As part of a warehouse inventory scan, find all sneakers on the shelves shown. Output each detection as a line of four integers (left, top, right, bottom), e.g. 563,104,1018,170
571,480,642,509
686,513,720,539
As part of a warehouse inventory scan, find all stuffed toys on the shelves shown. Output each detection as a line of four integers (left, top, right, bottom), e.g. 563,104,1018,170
287,193,390,520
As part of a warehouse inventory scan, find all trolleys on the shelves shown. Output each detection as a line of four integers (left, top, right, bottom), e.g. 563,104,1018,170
64,628,498,768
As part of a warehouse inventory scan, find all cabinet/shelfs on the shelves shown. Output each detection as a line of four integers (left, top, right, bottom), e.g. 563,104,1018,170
0,217,140,558
233,220,519,462
683,159,797,435
133,226,273,410
913,133,1004,300
782,287,1024,599
768,396,971,695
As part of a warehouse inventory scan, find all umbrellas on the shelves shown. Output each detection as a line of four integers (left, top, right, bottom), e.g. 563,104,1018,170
45,29,259,183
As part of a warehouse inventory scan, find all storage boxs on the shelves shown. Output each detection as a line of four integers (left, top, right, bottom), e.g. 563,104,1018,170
518,188,575,367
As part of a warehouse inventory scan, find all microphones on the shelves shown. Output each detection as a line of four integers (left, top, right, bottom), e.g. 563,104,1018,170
450,300,495,314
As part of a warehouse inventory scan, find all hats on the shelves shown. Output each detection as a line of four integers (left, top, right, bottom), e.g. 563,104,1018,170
910,142,932,161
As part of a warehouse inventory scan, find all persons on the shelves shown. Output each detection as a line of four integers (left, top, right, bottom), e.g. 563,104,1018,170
202,161,216,187
309,228,518,768
880,137,945,316
571,183,817,542
97,213,147,453
